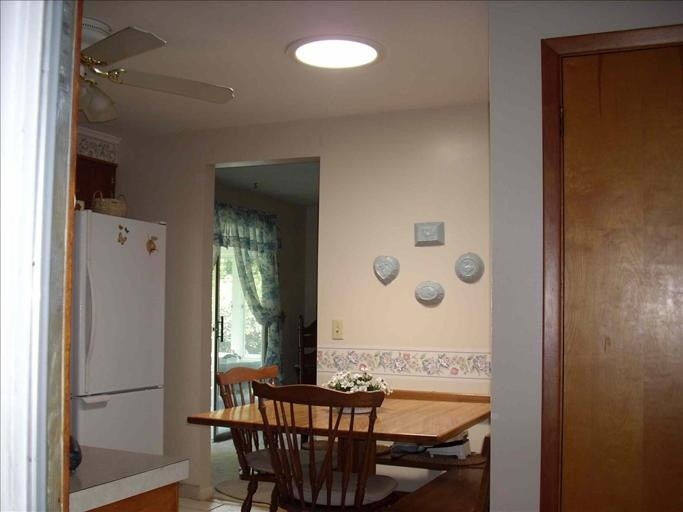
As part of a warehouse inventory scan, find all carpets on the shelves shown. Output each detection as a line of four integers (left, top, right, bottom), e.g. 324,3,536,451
215,478,276,505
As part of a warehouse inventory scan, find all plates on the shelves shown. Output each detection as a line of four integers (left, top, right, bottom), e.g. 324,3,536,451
413,279,444,306
412,222,444,247
454,252,486,283
372,254,400,285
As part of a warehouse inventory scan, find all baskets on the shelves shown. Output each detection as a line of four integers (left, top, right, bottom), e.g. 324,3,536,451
92,191,128,217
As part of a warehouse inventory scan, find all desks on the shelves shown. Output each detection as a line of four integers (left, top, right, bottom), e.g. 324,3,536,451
69,444,189,511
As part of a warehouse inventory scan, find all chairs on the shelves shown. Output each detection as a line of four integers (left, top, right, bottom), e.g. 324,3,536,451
251,380,398,511
216,366,335,511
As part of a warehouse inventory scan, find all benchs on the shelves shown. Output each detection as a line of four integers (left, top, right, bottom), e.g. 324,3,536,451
301,390,490,469
389,435,489,511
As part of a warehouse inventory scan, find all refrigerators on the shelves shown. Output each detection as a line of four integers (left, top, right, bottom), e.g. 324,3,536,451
71,206,165,462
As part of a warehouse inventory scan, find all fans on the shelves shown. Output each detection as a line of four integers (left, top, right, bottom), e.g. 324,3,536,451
76,17,234,123
